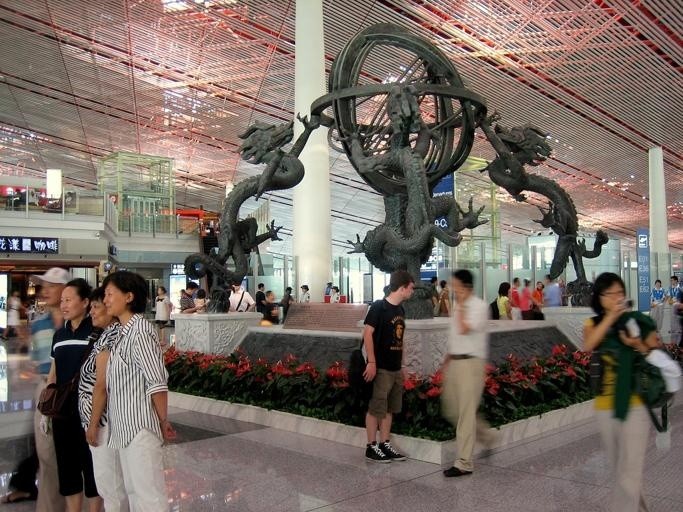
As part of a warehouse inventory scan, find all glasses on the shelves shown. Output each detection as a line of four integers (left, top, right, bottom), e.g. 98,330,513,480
599,291,625,298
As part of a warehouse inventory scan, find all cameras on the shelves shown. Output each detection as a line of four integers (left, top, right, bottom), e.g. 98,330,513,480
621,299,634,308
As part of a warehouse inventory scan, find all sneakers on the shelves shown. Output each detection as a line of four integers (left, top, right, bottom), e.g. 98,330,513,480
378,440,407,461
365,441,391,463
444,466,472,477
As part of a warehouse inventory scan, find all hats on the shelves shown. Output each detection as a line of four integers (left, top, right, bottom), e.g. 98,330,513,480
31,267,72,286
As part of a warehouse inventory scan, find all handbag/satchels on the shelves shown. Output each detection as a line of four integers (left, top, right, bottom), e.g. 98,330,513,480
348,350,373,402
491,301,499,320
36,383,75,416
636,361,674,409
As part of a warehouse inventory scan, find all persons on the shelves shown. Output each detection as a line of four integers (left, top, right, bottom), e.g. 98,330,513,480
0,435,39,504
330,286,340,304
649,280,667,308
32,268,121,512
179,281,208,313
664,276,683,303
438,269,497,477
2,289,29,339
102,272,177,512
431,276,442,317
440,280,452,317
154,286,173,344
255,282,311,326
498,274,572,321
582,272,662,512
362,270,416,463
229,282,256,312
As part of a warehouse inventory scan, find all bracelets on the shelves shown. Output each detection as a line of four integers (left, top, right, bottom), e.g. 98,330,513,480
367,362,376,363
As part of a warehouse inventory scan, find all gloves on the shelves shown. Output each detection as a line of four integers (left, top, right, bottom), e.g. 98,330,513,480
39,413,51,437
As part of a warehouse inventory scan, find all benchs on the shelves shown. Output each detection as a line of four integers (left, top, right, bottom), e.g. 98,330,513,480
60,193,76,209
9,191,26,204
